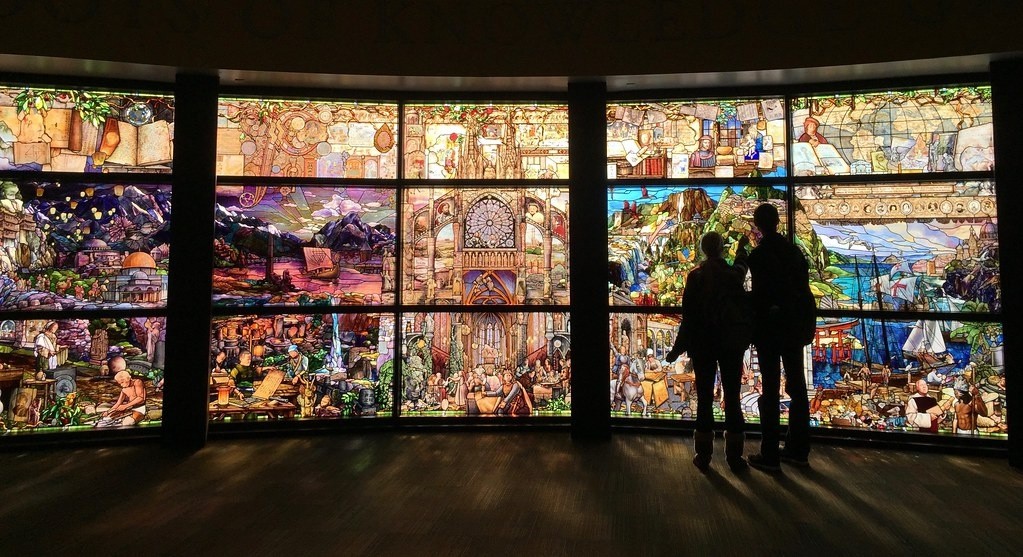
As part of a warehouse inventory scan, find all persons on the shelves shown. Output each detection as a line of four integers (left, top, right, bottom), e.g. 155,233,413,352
665,231,747,472
732,204,816,470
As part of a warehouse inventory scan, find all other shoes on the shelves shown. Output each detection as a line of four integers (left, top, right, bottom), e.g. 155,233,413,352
778,445,808,465
748,453,780,472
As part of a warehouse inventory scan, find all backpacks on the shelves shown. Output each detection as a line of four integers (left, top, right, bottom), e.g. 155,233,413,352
718,279,752,345
780,288,815,341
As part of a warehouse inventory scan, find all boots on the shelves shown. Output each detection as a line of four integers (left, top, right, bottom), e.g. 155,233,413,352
692,429,715,469
723,430,746,468
